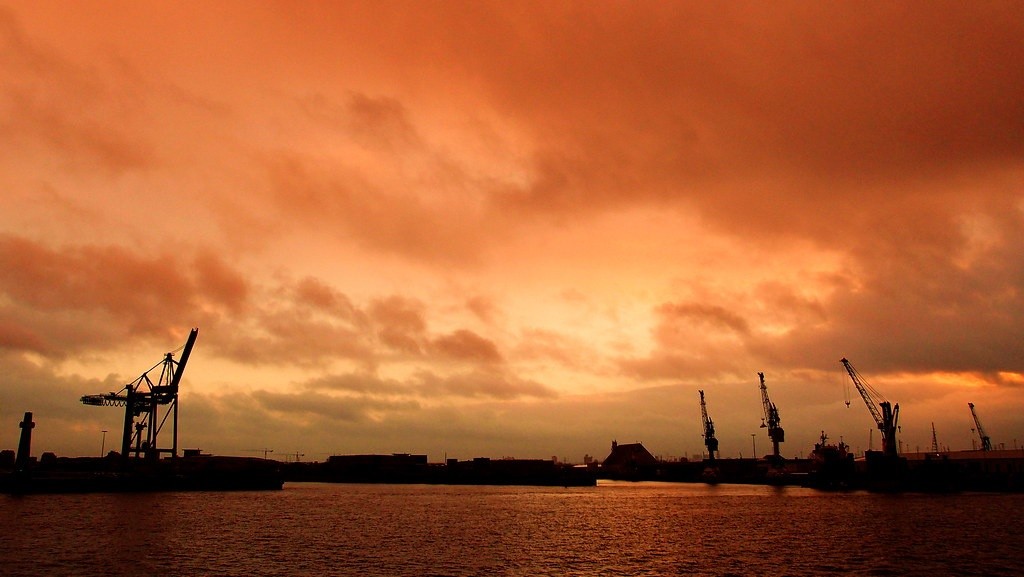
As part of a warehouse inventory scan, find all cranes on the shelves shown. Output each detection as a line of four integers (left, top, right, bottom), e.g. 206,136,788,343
756,371,785,455
931,421,939,453
241,447,305,463
966,402,994,452
840,355,900,462
868,427,1021,453
698,389,719,460
78,329,197,459
